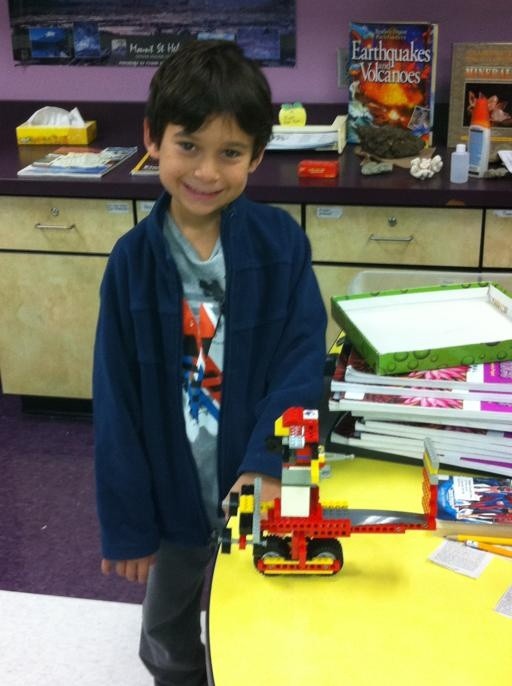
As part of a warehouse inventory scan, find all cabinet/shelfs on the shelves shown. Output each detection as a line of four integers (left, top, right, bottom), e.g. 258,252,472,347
0,198,135,404
484,209,512,296
306,205,482,362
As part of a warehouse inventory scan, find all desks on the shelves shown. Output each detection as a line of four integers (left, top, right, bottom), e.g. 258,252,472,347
197,443,512,686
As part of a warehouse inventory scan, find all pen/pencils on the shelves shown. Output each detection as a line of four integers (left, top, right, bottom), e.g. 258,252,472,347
442,534,512,545
466,540,512,559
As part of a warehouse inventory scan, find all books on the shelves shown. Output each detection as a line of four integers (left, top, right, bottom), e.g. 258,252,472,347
130,152,160,178
326,342,512,549
344,20,438,150
15,143,137,179
445,41,511,153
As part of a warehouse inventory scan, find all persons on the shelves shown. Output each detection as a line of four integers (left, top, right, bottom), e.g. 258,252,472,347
92,38,333,685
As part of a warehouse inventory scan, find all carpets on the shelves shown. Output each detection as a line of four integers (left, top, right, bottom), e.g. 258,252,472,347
0,407,211,612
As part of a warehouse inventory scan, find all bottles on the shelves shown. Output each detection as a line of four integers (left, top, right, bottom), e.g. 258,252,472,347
450,143,470,183
467,96,490,178
